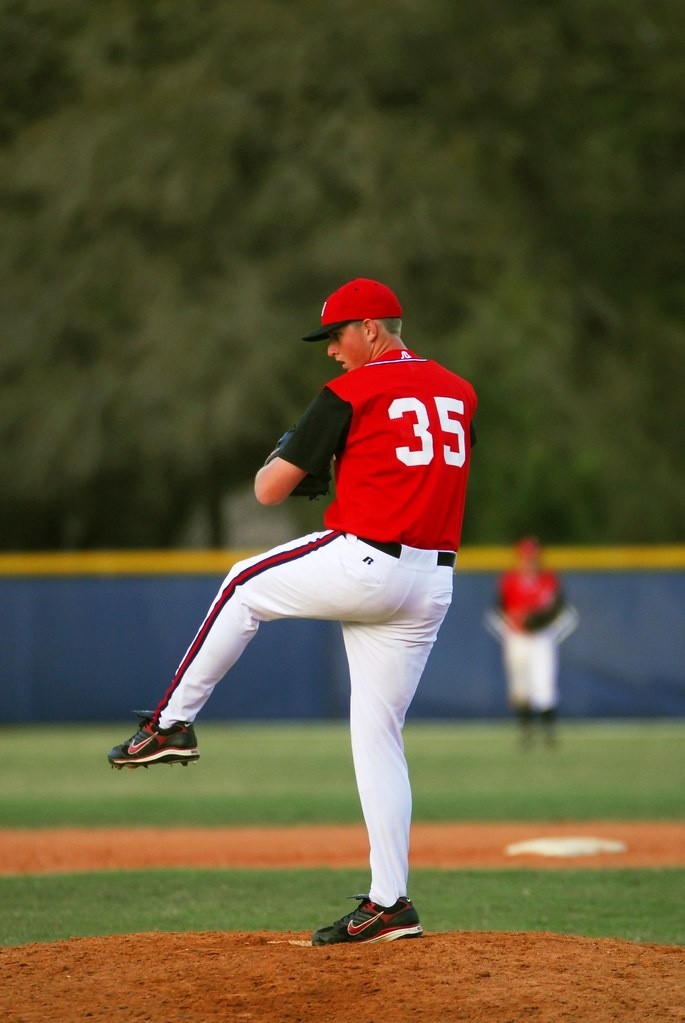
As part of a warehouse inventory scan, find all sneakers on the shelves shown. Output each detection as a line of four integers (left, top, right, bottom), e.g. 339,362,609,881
312,894,423,946
107,710,201,769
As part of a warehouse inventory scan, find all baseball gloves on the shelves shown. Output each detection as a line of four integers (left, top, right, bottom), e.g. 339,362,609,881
269,429,330,496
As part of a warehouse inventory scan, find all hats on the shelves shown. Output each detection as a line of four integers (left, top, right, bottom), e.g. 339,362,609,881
302,277,402,342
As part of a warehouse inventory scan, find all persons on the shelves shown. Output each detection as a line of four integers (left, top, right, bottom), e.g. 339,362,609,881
484,537,582,753
108,277,478,947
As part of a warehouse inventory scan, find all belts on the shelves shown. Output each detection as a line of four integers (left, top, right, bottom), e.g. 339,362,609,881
358,536,456,568
